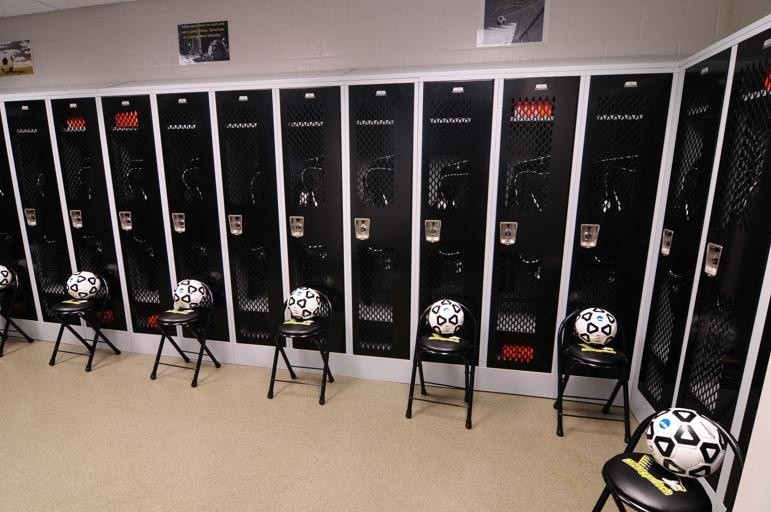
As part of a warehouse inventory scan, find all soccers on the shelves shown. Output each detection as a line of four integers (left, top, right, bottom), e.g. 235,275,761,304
429,299,464,335
496,15,506,25
647,407,725,478
66,271,100,300
174,279,206,309
0,53,14,74
288,287,320,320
208,40,226,59
0,265,12,290
575,308,618,348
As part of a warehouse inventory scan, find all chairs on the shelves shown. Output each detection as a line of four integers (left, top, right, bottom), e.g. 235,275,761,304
1,262,35,357
266,288,338,405
147,279,223,388
402,301,479,430
46,271,122,373
588,405,735,511
554,307,633,443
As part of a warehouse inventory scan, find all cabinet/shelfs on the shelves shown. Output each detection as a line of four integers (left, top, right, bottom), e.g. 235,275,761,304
0,99,43,343
1,62,86,356
553,60,683,405
689,13,771,512
208,62,291,370
95,60,182,358
413,62,501,392
479,61,587,402
150,61,234,363
341,61,421,386
45,61,132,354
626,35,739,439
273,60,350,378
